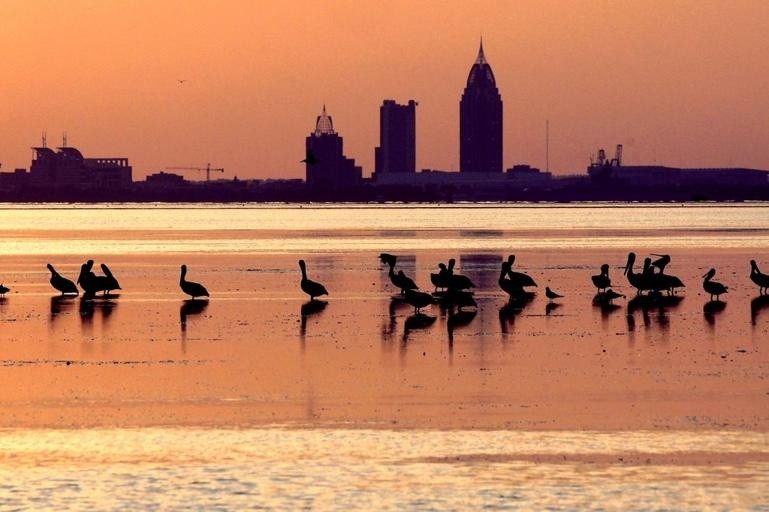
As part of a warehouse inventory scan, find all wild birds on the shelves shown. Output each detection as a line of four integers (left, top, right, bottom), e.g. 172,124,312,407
431,258,476,293
498,255,537,300
592,264,612,293
378,253,419,294
299,260,328,300
702,269,728,298
546,287,564,298
624,252,686,297
47,264,79,296
77,260,122,295
397,271,436,312
180,265,209,299
750,260,769,293
0,284,10,295
